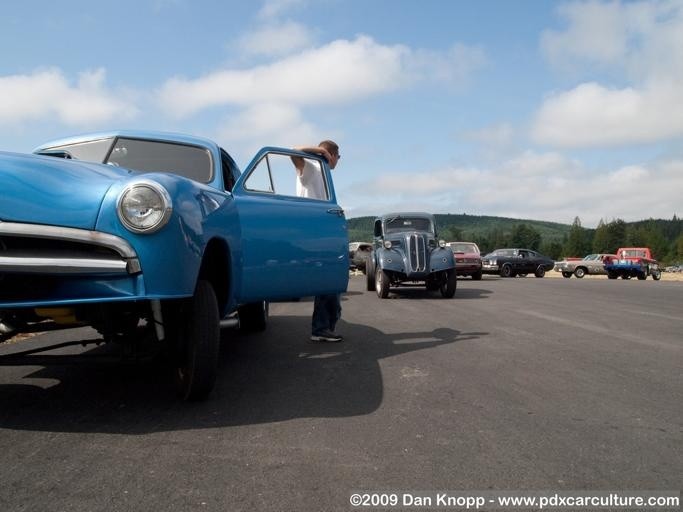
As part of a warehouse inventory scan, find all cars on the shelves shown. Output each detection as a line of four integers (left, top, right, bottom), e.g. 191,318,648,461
0,130,349,403
349,211,456,299
482,248,555,278
554,254,616,278
446,242,485,280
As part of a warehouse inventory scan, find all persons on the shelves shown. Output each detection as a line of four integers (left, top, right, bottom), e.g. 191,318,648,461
290,139,344,343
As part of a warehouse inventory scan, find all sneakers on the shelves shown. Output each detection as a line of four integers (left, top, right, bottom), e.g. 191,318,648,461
310,322,343,342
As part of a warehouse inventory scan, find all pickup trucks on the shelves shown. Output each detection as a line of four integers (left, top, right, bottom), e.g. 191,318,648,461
601,248,662,280
